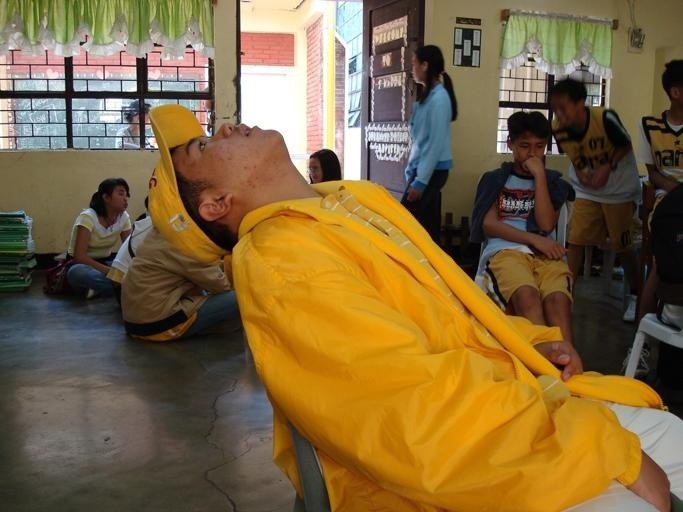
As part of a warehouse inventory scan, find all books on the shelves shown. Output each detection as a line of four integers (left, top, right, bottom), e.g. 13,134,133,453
0,212,37,288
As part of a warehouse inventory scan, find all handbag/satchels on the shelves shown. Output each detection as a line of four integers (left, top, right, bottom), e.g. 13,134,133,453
42,263,68,296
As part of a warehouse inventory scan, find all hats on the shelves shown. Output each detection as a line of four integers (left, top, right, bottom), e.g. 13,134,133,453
148,105,231,264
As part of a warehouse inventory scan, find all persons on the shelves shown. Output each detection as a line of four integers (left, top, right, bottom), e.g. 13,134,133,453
115,98,153,149
201,87,213,137
401,45,458,246
63,178,243,340
619,61,683,376
638,182,683,415
470,110,576,342
147,105,683,512
548,78,643,321
308,148,341,184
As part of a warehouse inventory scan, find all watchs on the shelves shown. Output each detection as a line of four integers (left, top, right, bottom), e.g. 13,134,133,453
607,159,617,170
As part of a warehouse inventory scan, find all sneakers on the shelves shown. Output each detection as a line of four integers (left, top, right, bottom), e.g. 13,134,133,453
624,348,649,377
